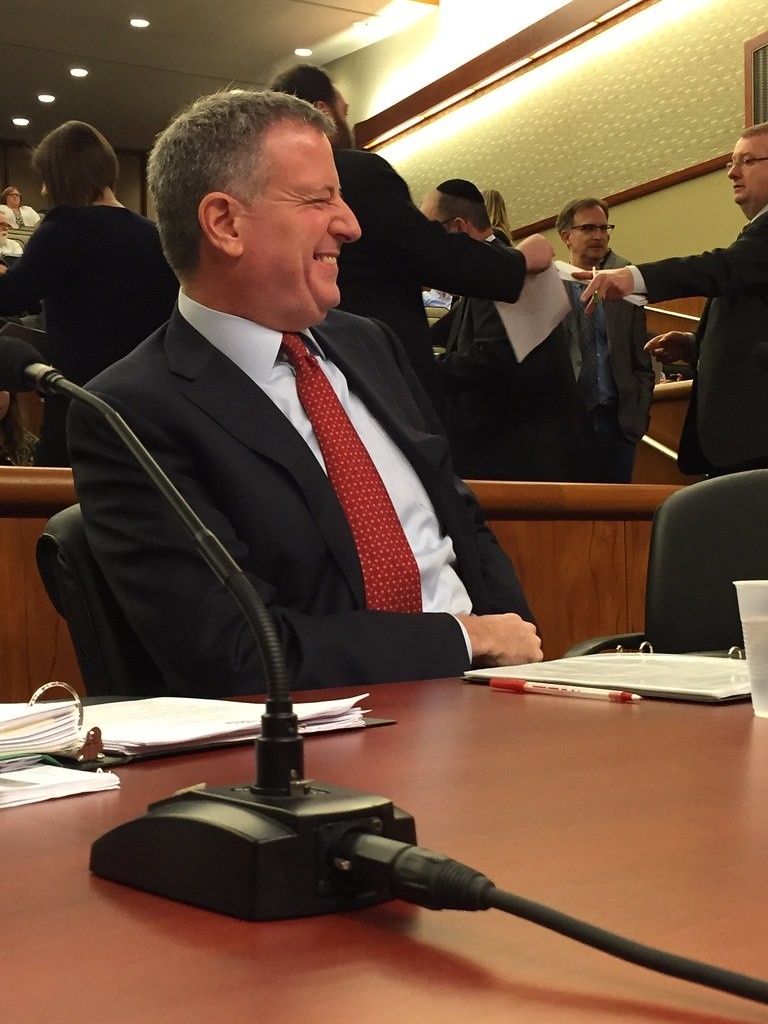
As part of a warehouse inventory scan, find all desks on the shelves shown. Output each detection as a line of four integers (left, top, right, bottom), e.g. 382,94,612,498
0,652,768,1024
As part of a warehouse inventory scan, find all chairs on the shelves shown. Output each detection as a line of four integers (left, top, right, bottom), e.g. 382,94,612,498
559,470,768,658
36,502,161,698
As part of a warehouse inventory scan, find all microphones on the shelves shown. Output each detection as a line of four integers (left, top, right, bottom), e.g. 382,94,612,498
0,332,419,924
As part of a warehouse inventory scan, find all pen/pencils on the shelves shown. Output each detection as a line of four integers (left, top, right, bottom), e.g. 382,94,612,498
489,677,643,705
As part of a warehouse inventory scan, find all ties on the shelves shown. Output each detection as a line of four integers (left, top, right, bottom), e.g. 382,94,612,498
278,333,423,614
576,283,601,417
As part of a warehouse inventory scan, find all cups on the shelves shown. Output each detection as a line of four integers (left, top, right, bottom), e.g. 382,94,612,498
733,579,768,719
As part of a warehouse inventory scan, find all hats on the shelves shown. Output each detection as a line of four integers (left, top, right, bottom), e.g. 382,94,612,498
0,205,19,230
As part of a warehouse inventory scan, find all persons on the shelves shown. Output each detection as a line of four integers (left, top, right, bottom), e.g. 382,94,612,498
419,179,573,483
0,391,39,466
271,62,556,428
572,119,768,484
0,187,40,273
66,93,544,697
558,197,656,483
0,121,181,464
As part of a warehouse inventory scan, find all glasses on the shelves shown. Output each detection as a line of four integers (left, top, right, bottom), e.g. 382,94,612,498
8,193,19,197
570,223,616,236
726,157,768,168
